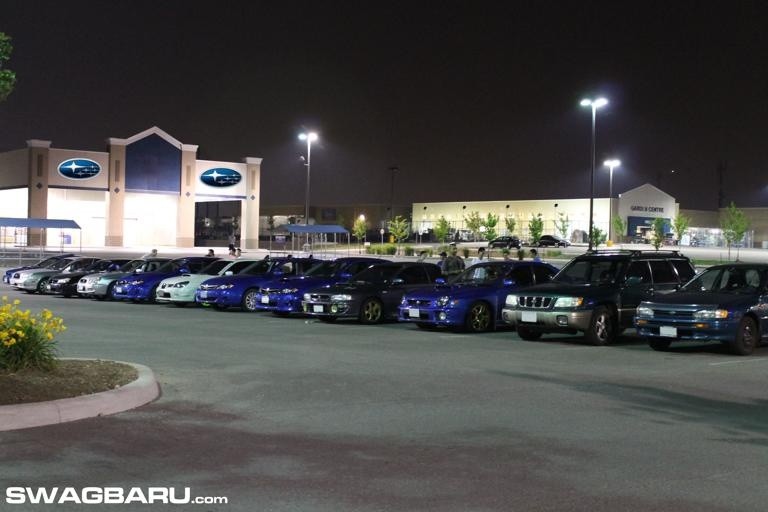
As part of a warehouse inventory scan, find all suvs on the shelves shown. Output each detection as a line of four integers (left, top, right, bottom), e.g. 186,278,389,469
505,248,699,342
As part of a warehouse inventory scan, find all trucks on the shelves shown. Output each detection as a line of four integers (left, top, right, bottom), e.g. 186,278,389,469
232,214,317,240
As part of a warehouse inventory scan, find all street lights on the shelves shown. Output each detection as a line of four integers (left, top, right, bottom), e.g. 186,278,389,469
580,95,609,254
297,130,321,247
412,202,565,238
379,229,385,250
388,166,396,223
601,157,621,253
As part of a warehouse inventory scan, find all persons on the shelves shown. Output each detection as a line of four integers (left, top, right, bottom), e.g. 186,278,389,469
413,244,542,281
139,226,314,262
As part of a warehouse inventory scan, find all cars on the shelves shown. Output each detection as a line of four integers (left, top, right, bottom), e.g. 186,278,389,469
633,261,767,354
303,260,441,328
3,253,130,298
157,258,260,306
114,259,213,304
198,258,292,310
398,262,556,337
78,257,160,299
256,257,393,315
488,236,522,250
538,234,570,247
